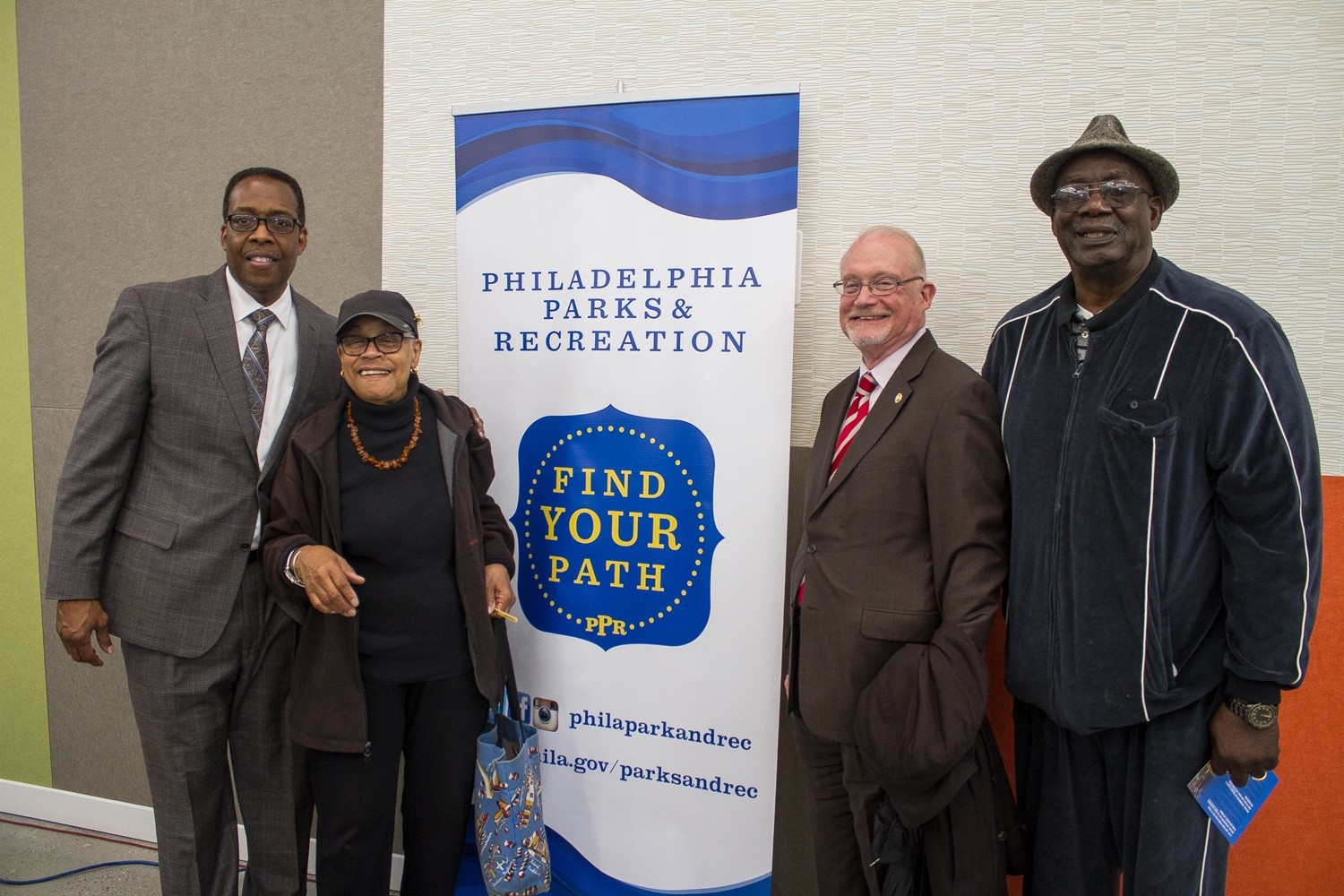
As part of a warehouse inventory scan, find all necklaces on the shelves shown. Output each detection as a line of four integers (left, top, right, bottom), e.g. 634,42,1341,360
346,396,422,471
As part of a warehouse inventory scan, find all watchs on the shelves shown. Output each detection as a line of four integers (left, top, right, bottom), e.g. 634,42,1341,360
1225,694,1279,729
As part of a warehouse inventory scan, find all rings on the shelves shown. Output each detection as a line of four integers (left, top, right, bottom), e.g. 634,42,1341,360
1251,770,1267,781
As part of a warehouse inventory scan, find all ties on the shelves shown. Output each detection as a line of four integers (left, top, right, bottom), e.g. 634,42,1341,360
242,309,276,448
797,371,879,606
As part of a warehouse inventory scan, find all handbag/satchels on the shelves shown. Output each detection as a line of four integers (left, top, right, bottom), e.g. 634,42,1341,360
475,616,551,896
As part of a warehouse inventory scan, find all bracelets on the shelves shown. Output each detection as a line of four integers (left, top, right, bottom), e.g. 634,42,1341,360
284,548,305,587
293,551,306,585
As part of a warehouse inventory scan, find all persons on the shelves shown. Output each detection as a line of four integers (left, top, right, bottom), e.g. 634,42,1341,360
980,113,1324,896
786,227,1008,896
45,167,484,896
261,291,516,896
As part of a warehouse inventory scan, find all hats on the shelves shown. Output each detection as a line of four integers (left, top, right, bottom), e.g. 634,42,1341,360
333,289,418,338
1030,114,1179,218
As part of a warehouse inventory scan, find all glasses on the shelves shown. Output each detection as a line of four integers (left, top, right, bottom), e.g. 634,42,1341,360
1051,180,1152,213
225,213,303,234
338,332,416,356
833,277,924,296
